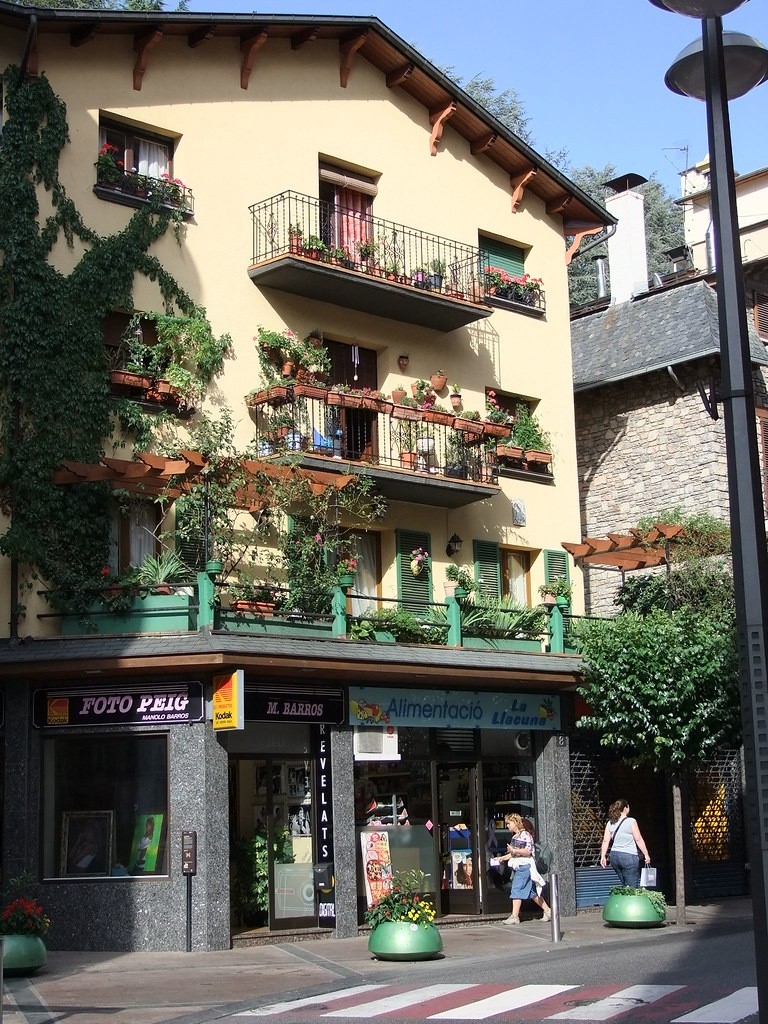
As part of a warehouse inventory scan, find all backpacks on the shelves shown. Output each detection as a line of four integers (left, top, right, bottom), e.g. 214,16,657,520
534,842,551,874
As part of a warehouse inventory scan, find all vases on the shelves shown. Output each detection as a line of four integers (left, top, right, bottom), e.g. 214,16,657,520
1,933,48,980
602,894,664,928
367,916,445,964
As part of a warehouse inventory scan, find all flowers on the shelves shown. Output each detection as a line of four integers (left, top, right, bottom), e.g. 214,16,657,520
0,868,56,940
363,868,438,931
610,884,669,916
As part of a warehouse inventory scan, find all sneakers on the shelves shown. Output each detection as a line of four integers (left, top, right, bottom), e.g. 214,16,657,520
539,908,553,923
502,915,520,925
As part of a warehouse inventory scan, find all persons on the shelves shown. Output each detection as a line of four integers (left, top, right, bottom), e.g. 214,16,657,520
491,810,554,925
452,853,474,888
68,816,108,872
599,798,653,889
130,815,154,870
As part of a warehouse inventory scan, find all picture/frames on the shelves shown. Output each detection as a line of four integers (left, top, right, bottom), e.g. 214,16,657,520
124,805,168,877
247,760,313,839
57,808,116,881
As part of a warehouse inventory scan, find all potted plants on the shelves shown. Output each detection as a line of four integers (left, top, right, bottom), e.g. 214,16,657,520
64,145,588,650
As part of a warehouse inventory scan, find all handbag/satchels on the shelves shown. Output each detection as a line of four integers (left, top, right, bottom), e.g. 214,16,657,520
640,863,657,887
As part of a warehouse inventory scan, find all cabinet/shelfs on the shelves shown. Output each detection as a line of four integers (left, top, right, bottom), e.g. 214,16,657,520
350,758,538,836
